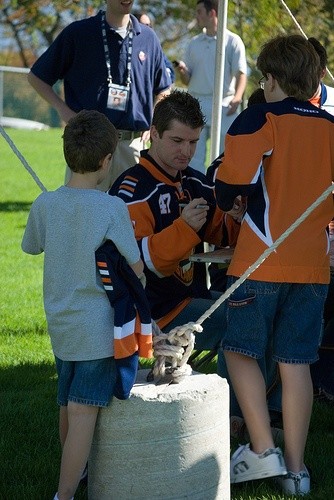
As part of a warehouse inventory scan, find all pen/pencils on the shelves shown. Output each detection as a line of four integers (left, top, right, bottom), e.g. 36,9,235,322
178,202,210,210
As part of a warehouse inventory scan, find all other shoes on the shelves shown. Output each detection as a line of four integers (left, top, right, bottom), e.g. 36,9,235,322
231,443,311,496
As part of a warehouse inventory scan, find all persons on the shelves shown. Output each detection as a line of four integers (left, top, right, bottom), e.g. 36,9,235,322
204,33,334,495
22,111,146,500
28,0,177,193
107,88,284,450
171,0,247,175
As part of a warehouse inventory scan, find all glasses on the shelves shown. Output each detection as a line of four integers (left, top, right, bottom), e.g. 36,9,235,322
259,75,265,89
